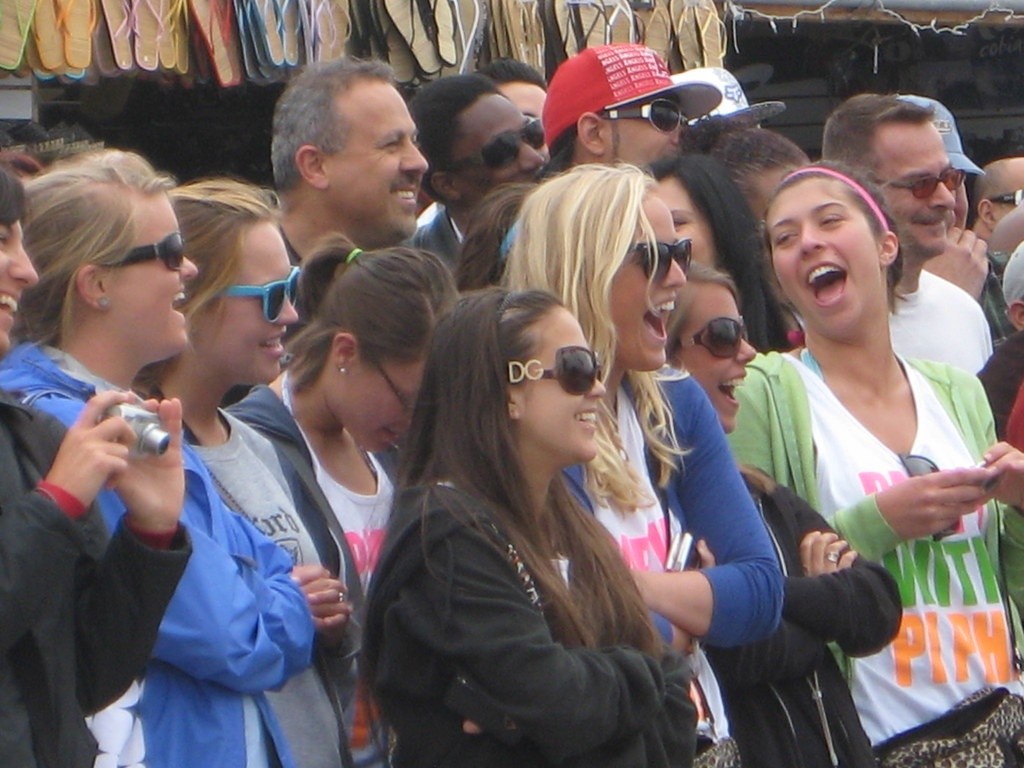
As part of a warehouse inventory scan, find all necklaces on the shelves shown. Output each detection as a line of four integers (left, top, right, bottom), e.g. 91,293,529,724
203,462,256,526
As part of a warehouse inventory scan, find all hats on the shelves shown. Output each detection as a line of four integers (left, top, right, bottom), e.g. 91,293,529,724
894,95,986,177
669,67,786,124
1002,240,1024,305
541,43,724,147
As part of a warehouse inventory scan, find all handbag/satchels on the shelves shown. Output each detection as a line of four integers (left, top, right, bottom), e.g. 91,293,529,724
870,681,1024,768
688,738,744,768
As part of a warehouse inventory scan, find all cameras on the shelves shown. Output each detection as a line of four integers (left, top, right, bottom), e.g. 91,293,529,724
976,461,999,493
98,394,172,458
664,533,702,575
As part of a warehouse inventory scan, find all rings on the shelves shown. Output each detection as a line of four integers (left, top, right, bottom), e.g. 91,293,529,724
338,593,343,602
824,552,839,563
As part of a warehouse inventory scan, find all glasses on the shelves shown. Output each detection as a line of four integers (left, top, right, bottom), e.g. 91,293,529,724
448,118,546,180
626,237,693,284
901,451,958,542
102,231,184,271
681,316,748,358
598,97,693,133
207,266,300,322
507,345,602,396
988,189,1024,207
866,169,967,198
360,341,417,417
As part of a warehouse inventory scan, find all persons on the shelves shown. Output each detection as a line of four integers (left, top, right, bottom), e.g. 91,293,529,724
0,53,439,767
356,46,783,768
725,92,1024,768
